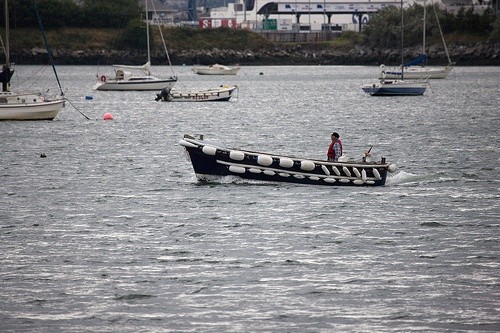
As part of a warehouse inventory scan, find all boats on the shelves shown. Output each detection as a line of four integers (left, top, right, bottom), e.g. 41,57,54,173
179,133,396,187
362,73,431,96
191,64,240,75
154,85,239,102
380,65,454,78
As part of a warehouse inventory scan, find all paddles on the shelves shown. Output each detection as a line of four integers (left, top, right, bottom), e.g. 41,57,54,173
362,146,373,163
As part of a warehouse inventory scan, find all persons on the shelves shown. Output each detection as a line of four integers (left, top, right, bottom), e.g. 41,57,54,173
327,132,342,162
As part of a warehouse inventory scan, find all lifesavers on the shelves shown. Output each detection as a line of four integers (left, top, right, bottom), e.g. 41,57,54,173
100,75,106,82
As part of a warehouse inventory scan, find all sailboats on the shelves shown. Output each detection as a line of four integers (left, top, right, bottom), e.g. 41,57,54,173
93,0,178,90
0,0,91,121
